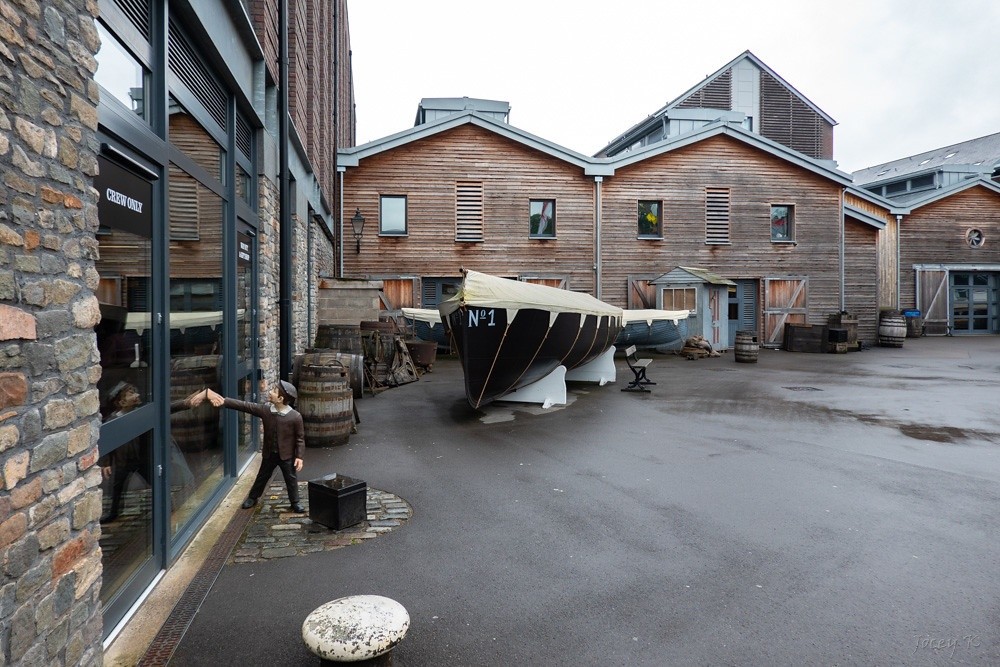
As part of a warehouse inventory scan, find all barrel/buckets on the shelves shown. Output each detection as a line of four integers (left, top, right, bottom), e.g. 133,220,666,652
292,354,364,398
905,317,922,337
298,364,354,448
878,309,907,347
735,330,758,363
305,321,395,366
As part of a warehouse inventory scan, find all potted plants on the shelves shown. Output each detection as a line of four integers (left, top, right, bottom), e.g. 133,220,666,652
829,310,858,344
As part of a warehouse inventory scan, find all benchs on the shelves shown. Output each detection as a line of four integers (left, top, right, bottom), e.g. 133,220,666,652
620,344,657,393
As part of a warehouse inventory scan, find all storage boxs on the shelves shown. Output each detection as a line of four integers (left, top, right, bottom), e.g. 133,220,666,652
309,473,367,530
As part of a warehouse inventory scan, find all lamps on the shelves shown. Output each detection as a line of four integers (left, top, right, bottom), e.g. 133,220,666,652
351,206,365,253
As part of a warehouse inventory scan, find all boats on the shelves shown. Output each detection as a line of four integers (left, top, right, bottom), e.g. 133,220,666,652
126,309,244,370
438,267,626,410
402,307,689,356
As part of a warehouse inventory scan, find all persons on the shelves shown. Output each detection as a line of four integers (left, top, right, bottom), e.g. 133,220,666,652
207,380,305,513
99,380,206,524
